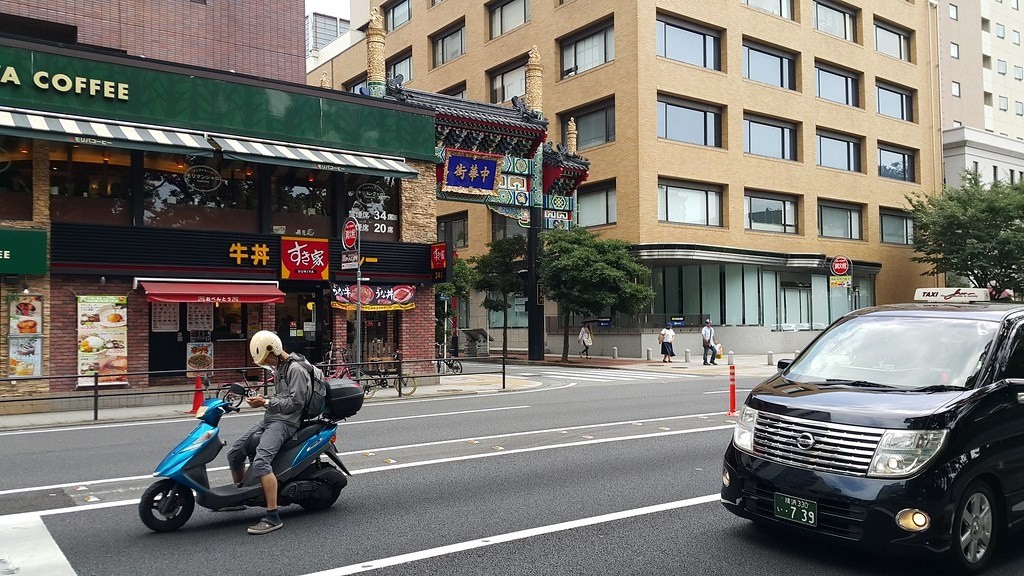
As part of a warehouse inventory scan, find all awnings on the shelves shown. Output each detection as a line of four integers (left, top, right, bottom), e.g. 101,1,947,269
0,110,419,182
141,282,285,303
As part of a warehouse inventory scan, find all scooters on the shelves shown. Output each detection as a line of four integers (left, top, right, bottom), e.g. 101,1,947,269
139,374,364,531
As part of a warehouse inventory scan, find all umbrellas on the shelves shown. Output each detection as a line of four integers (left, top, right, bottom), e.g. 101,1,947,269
580,316,599,323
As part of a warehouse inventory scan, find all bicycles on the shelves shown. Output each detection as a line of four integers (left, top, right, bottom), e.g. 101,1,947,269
216,370,274,408
435,342,462,375
314,342,417,400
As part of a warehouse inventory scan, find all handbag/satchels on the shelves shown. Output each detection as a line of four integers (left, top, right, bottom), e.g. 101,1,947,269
715,344,722,360
658,335,664,342
584,338,593,346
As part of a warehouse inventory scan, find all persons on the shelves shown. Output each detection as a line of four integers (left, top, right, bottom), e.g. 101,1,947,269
577,322,591,359
210,330,307,534
701,319,718,365
659,323,676,362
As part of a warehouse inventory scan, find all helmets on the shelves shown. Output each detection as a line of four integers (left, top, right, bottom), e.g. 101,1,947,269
250,331,282,364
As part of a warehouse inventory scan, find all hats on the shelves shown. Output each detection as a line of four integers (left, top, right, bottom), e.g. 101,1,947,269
707,319,712,324
666,322,673,329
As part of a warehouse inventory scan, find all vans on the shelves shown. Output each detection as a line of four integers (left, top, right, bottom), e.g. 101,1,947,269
721,288,1024,576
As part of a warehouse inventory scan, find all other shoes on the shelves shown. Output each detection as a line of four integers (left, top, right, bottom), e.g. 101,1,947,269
579,352,583,358
586,357,592,359
668,360,672,363
663,360,667,363
704,360,709,365
710,360,717,365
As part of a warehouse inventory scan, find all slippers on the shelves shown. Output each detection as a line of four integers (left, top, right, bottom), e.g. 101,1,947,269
248,516,282,534
209,506,250,511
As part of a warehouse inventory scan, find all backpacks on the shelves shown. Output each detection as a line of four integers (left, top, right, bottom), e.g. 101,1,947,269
283,356,326,420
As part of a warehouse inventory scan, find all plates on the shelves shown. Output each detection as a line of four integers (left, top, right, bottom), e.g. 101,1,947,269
187,353,213,370
97,308,127,327
